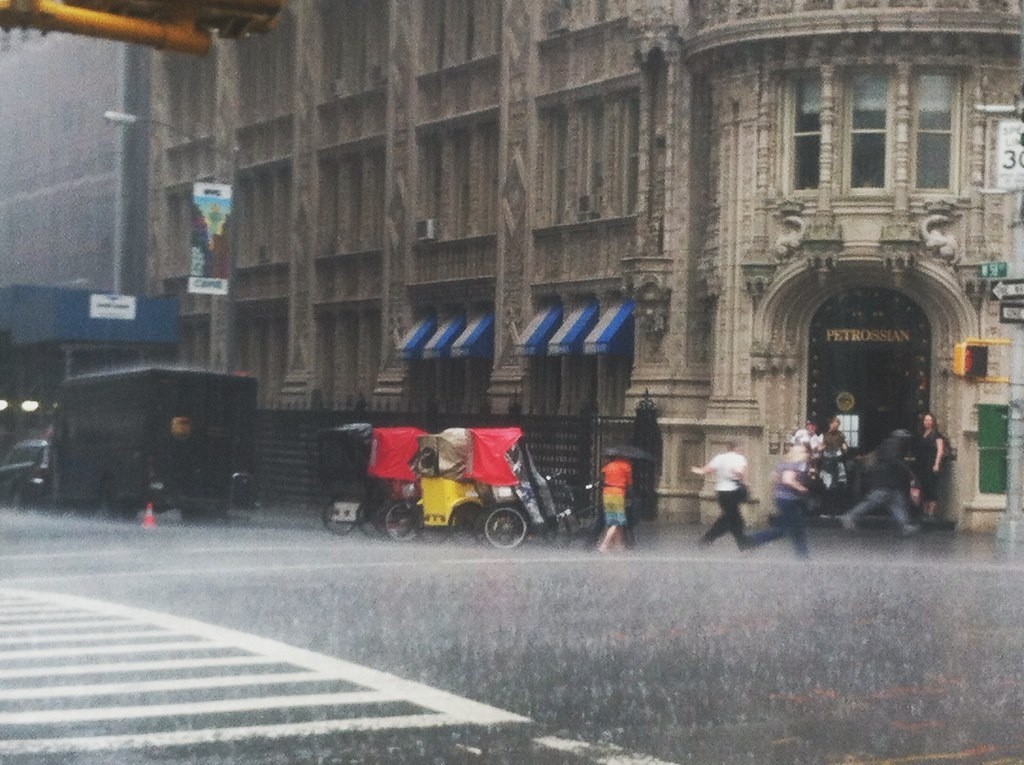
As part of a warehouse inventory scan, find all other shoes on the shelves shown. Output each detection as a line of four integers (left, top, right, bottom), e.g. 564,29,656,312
901,523,923,538
836,515,857,534
598,546,614,558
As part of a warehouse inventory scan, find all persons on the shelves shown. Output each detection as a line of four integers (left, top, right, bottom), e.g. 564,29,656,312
601,456,632,552
793,415,919,534
916,411,944,524
747,446,810,557
690,438,753,548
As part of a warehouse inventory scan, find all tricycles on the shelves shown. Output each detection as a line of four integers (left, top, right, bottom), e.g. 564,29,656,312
323,420,572,548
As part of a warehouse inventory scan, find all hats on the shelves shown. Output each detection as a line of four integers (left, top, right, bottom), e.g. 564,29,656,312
806,418,817,425
893,428,912,437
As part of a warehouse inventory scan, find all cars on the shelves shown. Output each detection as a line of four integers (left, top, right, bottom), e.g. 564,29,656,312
0,439,55,508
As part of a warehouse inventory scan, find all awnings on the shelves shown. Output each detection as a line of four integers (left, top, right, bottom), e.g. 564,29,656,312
449,309,495,356
422,310,465,359
396,312,435,360
512,302,562,357
547,299,599,354
584,299,634,354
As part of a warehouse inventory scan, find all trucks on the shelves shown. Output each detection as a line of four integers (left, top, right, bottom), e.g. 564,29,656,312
56,366,261,519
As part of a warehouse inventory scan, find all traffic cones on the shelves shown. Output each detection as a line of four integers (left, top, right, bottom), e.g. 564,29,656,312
139,501,164,533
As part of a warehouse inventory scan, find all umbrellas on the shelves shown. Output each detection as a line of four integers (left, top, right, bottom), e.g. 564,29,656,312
605,443,657,461
